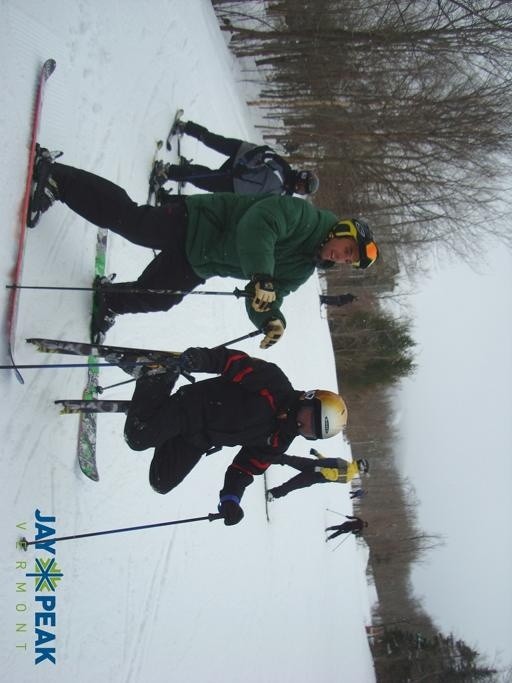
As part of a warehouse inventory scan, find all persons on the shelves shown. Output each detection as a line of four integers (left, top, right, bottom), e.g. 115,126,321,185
28,116,382,545
